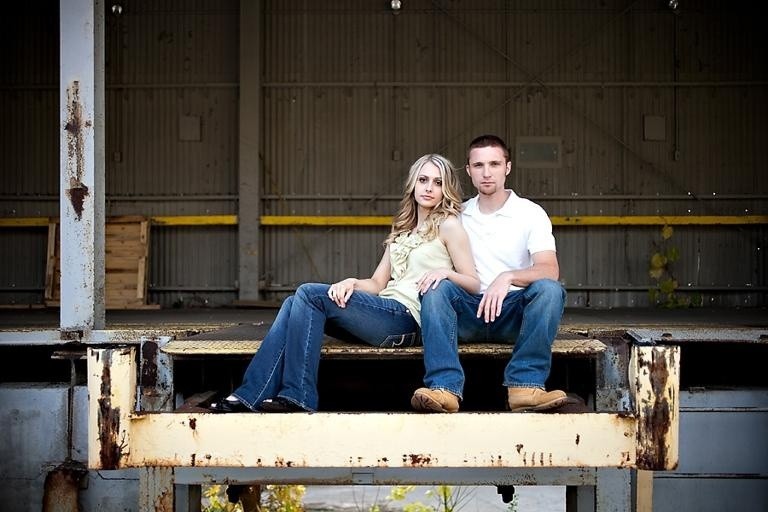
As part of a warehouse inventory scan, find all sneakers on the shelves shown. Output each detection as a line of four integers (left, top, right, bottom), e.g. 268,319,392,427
508,387,569,413
411,387,459,412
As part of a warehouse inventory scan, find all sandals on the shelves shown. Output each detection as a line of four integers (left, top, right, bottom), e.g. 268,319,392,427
255,397,307,413
209,398,240,411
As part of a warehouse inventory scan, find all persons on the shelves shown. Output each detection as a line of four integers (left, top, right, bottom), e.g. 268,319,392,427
410,134,569,413
210,152,481,412
